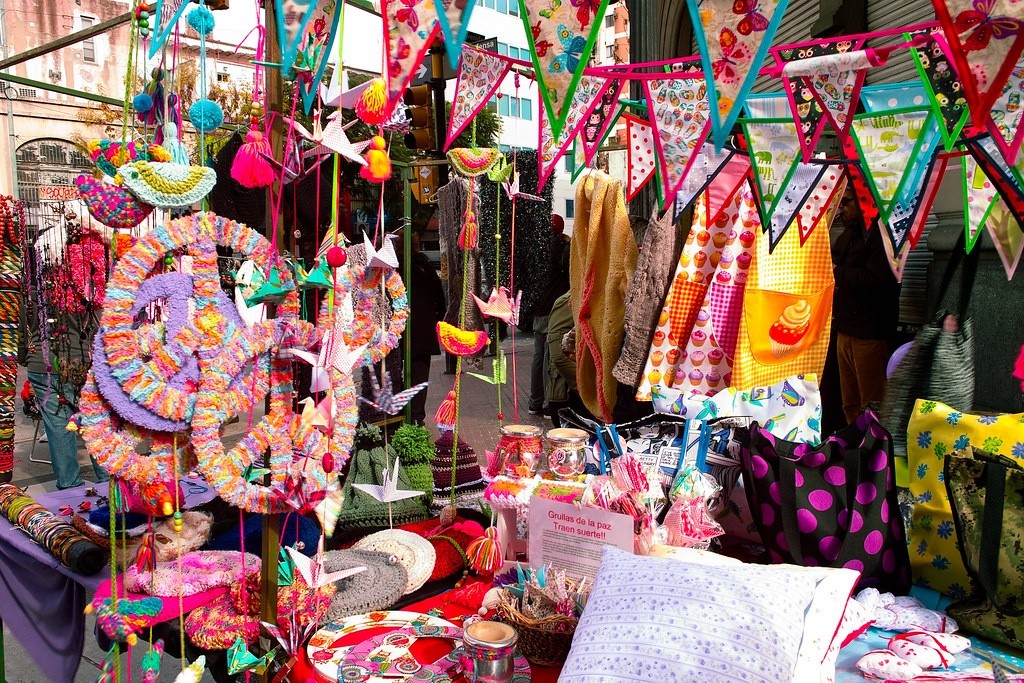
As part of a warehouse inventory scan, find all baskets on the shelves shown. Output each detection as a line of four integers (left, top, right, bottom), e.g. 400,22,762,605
499,577,588,667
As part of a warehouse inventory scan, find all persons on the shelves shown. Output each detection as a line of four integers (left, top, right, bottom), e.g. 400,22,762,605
832,186,899,425
394,229,446,426
27,233,111,491
530,215,576,428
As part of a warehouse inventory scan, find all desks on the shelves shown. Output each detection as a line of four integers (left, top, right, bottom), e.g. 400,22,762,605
137,613,239,683
0,480,120,601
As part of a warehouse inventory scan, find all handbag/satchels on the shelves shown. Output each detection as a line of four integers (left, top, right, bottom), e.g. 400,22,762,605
902,398,1024,658
712,406,914,595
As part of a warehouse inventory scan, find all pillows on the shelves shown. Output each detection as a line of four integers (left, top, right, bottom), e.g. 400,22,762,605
558,545,830,683
647,542,862,683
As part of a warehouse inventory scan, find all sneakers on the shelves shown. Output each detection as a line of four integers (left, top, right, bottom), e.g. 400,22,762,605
527,407,551,420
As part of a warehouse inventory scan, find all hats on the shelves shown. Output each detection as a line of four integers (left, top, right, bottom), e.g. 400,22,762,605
338,420,429,530
431,422,485,499
390,422,438,509
